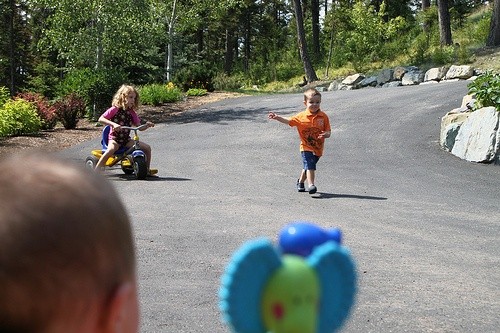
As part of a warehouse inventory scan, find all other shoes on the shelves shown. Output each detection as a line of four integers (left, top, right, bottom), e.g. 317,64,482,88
146,168,158,176
296,179,305,192
308,185,317,194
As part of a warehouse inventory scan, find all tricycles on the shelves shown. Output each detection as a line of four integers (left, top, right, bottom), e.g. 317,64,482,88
85,122,153,180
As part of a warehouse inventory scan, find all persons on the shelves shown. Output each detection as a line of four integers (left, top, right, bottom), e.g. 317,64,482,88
267,86,331,193
92,83,158,177
1,143,141,333
